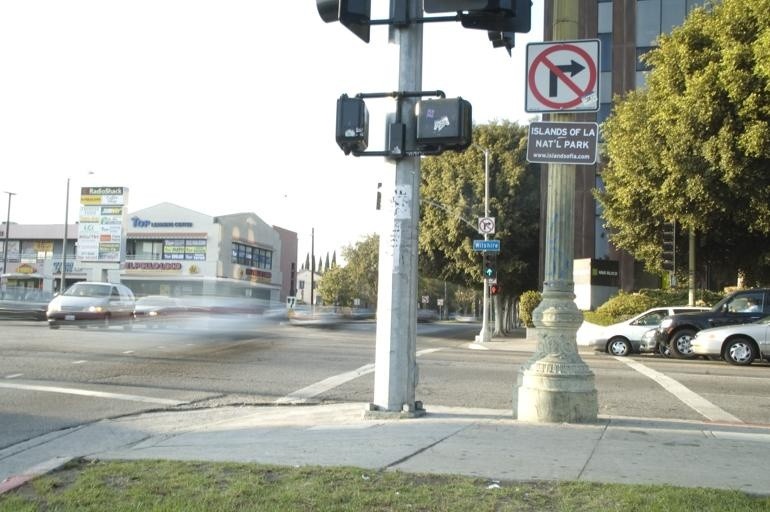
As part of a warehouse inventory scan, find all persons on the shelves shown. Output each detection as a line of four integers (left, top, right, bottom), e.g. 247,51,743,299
641,311,666,325
728,295,760,313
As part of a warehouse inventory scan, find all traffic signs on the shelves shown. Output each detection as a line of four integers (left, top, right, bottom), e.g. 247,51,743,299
473,240,500,251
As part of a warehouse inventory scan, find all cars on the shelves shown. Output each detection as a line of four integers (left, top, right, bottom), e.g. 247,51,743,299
594,288,770,365
418,309,458,323
1,287,53,320
46,281,136,329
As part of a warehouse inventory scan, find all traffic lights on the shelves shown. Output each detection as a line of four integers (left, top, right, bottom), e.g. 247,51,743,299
661,218,675,274
483,251,497,279
492,285,498,295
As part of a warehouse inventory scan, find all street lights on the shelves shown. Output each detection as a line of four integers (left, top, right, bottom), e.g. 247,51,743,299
59,172,95,294
